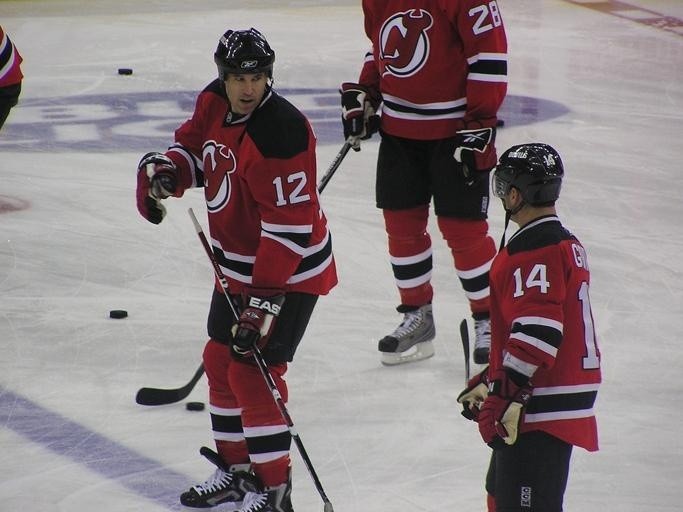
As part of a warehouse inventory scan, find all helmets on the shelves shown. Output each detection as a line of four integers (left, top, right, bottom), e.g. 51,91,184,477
492,143,564,204
213,28,275,82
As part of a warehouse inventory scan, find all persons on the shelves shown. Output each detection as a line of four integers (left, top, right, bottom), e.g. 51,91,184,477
338,0,510,364
0,18,27,137
454,142,603,512
135,26,337,512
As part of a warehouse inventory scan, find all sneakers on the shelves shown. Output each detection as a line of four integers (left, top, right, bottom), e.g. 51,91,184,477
472,311,491,364
378,301,436,352
180,446,255,508
234,466,294,512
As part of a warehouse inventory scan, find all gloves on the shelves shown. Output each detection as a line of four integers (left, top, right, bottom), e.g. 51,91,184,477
478,370,534,449
457,365,490,421
230,284,286,359
339,82,380,152
136,151,180,224
453,115,497,178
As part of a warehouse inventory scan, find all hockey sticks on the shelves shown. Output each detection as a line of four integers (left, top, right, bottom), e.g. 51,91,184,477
460,318,504,436
188,208,332,511
136,140,352,406
